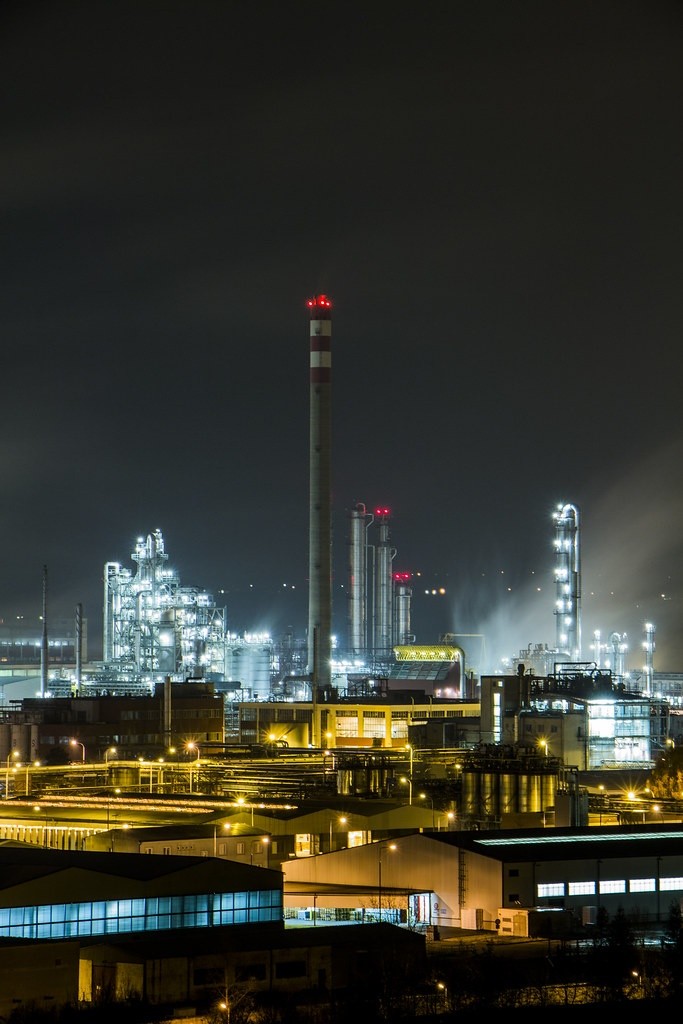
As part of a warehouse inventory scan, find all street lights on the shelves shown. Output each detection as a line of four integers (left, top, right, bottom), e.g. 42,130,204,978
406,743,414,775
328,817,348,854
187,742,201,760
105,747,116,765
400,776,413,805
420,793,435,832
70,739,86,764
237,797,254,827
5,750,20,799
454,764,462,779
168,748,180,765
107,788,122,829
25,760,41,797
